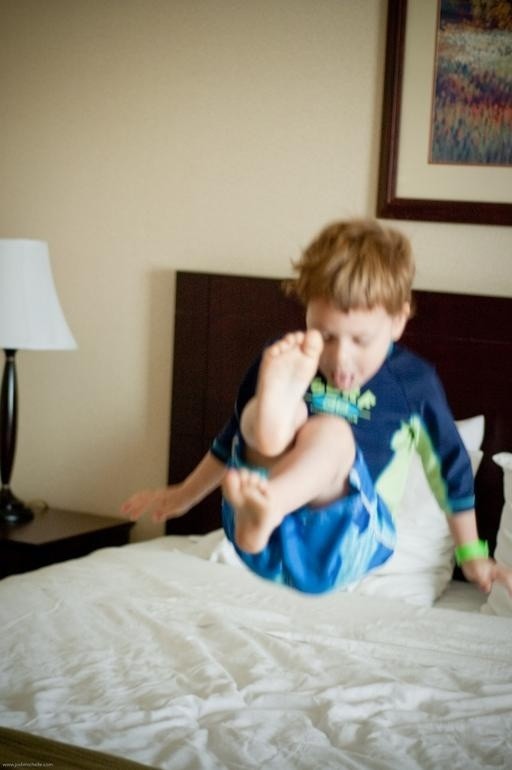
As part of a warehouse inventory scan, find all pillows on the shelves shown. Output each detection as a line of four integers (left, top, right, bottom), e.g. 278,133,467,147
479,453,512,620
190,403,487,611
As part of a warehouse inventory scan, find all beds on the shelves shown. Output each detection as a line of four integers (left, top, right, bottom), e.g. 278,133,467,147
0,268,512,768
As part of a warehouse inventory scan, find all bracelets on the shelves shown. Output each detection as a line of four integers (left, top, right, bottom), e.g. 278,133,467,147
453,540,489,567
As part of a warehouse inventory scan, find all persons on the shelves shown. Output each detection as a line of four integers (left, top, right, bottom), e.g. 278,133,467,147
120,219,512,594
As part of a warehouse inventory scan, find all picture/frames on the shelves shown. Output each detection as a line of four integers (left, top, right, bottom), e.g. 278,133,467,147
375,2,511,223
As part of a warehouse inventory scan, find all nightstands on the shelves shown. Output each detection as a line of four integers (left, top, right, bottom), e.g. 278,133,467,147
0,499,138,572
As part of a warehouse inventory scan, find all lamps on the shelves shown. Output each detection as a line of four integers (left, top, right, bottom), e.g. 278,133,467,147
2,236,76,533
